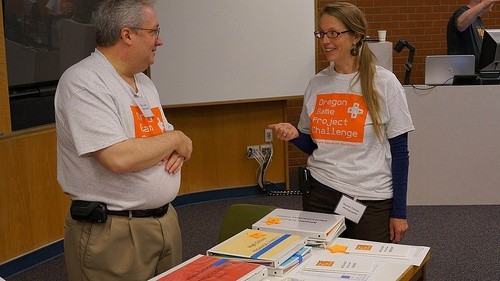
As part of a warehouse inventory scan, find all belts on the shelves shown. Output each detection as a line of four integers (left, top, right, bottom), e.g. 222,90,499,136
107,203,169,218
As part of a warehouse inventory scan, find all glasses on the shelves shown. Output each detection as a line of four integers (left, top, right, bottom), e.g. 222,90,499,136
129,27,160,35
314,30,350,38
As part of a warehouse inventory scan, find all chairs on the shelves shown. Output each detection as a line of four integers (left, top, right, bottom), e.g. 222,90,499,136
218,204,275,243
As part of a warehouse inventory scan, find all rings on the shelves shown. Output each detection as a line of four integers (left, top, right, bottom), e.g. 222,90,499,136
282,134,286,136
178,158,182,161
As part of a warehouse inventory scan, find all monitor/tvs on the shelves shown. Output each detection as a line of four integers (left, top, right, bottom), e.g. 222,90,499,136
426,54,475,85
475,29,500,72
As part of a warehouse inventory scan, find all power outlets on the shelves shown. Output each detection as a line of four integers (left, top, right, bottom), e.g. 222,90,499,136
247,145,259,159
265,129,273,142
260,145,273,157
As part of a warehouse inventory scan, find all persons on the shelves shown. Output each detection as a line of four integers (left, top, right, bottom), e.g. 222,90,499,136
54,0,192,281
447,0,494,64
268,2,415,243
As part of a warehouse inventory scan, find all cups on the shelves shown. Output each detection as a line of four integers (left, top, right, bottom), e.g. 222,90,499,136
377,30,386,43
